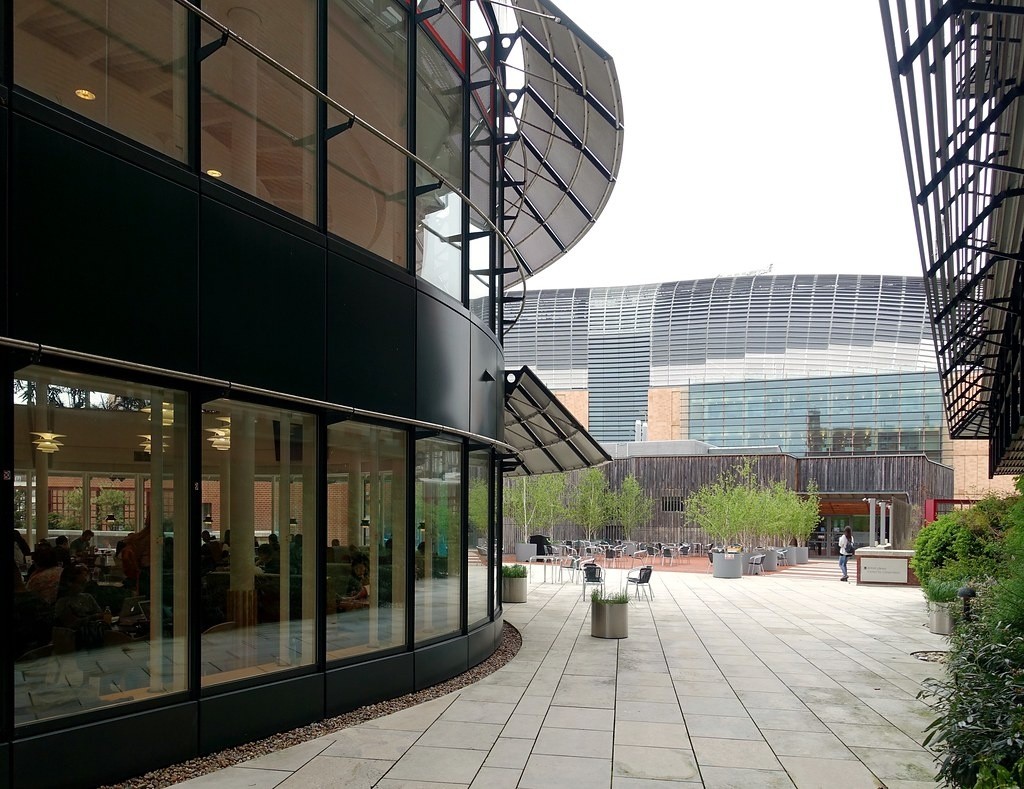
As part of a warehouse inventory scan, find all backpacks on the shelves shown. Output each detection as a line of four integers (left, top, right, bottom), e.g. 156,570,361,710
846,537,855,556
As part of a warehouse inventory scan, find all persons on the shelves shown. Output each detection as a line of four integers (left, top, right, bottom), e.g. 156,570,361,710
13,516,425,647
839,526,855,582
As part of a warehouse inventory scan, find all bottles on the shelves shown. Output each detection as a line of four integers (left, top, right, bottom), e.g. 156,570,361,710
103,606,111,624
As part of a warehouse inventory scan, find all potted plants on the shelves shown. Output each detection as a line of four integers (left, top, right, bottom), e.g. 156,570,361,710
675,454,824,579
922,579,965,636
502,563,528,603
589,589,631,639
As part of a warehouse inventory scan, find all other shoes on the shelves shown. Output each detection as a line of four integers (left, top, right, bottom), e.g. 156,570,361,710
841,575,849,581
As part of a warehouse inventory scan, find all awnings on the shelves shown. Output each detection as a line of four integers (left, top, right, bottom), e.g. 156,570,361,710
501,363,607,489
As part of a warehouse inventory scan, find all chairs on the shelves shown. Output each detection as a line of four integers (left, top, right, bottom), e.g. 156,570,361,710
543,539,788,601
51,625,76,655
100,629,131,650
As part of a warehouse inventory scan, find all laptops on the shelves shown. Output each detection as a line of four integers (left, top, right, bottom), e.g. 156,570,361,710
111,595,173,623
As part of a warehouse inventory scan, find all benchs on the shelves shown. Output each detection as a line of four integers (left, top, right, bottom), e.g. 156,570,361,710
207,545,444,625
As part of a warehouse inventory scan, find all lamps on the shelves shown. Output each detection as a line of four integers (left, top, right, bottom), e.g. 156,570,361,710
204,412,257,450
484,370,497,382
202,481,214,524
290,519,298,526
359,520,371,528
141,401,175,426
418,523,425,531
136,428,171,455
30,408,67,454
105,478,117,523
